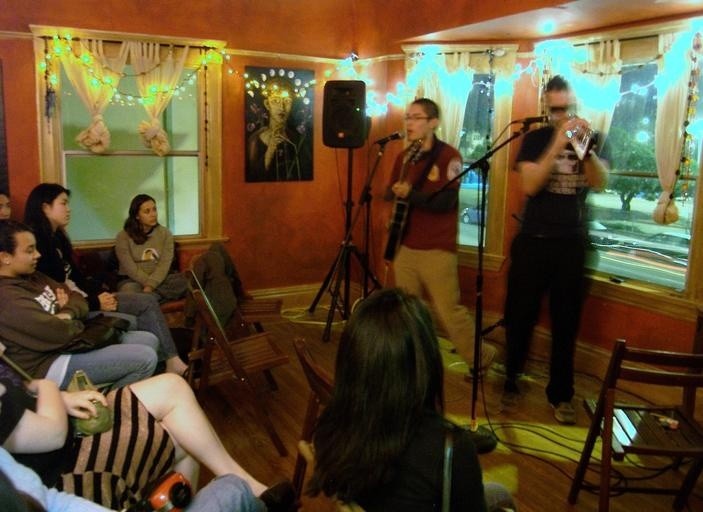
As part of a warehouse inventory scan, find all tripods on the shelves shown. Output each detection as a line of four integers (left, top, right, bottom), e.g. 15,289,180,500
308,148,383,342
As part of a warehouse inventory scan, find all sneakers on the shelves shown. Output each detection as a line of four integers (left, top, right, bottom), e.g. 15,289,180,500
547,397,578,426
464,349,499,383
499,388,524,414
258,479,300,512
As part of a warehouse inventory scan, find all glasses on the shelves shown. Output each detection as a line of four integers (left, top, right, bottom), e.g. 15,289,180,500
547,104,571,114
406,115,433,120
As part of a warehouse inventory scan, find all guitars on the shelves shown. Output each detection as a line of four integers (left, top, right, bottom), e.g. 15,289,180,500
383,139,423,261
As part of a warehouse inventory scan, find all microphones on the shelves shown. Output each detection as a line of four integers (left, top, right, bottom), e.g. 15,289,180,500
376,131,405,144
519,115,549,124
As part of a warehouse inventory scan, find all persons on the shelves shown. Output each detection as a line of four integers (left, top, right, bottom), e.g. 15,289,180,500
247,79,312,181
0,447,295,510
113,193,187,305
497,74,610,425
382,99,498,382
0,192,14,221
22,181,189,379
0,219,161,392
0,370,269,510
312,287,515,511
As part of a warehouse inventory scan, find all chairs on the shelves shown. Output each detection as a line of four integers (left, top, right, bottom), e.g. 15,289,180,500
173,259,291,464
290,333,337,510
203,234,284,336
567,327,701,511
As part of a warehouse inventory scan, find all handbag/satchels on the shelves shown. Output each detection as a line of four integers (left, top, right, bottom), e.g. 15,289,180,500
52,312,131,354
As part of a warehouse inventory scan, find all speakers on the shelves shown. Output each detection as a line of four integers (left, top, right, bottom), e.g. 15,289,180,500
322,80,366,149
